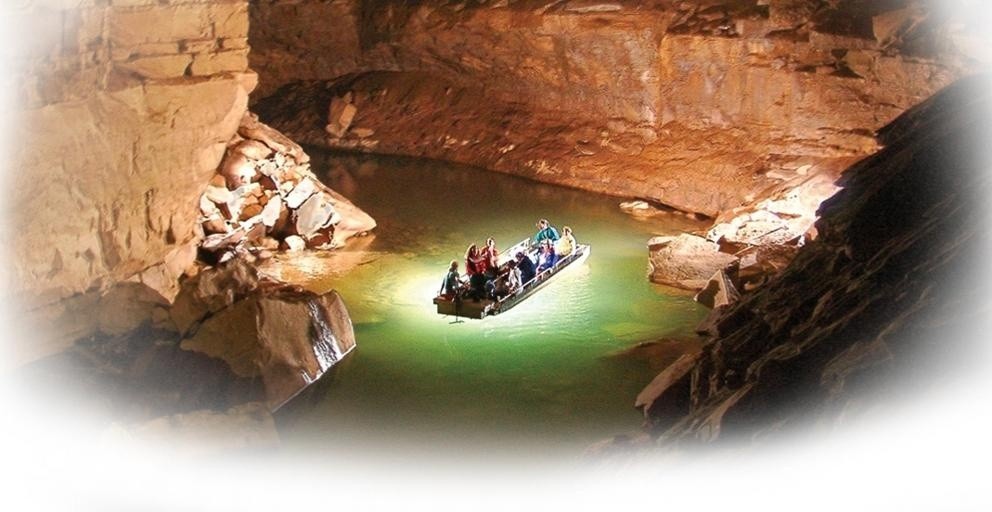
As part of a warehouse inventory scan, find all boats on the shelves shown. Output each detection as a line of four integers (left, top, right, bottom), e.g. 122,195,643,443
434,237,591,320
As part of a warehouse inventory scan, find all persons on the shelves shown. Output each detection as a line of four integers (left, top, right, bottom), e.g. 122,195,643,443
439,218,577,303
